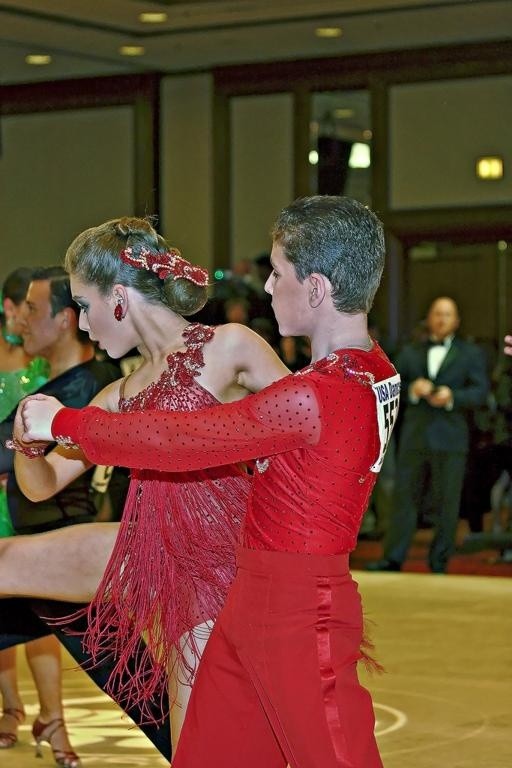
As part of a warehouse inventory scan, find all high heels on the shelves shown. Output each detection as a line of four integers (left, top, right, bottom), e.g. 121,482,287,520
31,716,82,768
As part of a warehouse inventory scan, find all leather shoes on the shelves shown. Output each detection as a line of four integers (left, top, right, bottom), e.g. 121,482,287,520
367,556,397,570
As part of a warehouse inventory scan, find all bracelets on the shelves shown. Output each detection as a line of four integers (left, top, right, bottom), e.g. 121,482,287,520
7,434,54,458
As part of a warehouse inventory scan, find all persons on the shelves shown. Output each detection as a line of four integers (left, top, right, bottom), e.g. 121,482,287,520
217,255,313,374
0,264,172,768
23,193,401,767
360,295,512,574
1,214,297,768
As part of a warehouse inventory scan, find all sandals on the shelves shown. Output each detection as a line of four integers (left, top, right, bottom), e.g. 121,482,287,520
0,706,27,750
